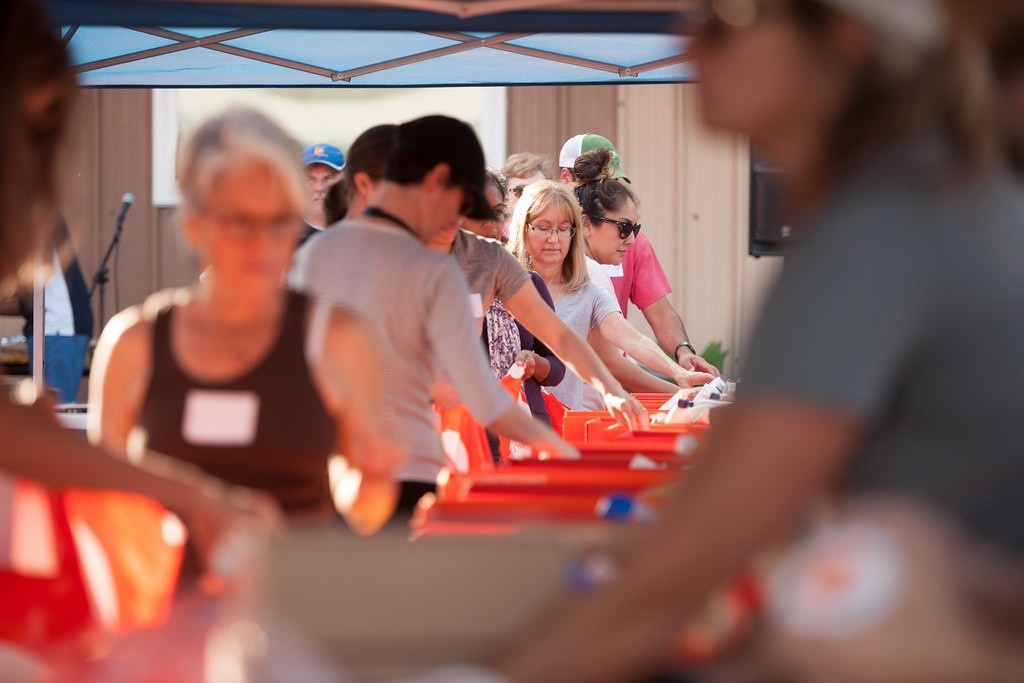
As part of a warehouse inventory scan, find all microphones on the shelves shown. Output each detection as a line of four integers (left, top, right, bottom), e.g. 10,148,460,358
116,191,135,225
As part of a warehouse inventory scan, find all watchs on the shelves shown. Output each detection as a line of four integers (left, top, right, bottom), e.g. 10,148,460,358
675,342,696,362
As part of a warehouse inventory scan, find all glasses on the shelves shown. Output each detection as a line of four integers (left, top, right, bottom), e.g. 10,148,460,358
508,184,524,197
527,222,576,239
582,212,642,239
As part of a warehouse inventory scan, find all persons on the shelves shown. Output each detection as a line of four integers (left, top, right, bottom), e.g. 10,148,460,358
512,1,1024,682
570,178,682,393
459,169,567,464
557,132,722,378
17,207,95,405
1,0,289,683
295,113,652,545
499,147,715,391
84,99,399,613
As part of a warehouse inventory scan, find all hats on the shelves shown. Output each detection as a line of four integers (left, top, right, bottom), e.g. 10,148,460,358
397,115,498,220
301,142,345,170
559,132,631,184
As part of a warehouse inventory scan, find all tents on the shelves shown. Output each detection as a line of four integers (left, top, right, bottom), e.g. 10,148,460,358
0,0,708,393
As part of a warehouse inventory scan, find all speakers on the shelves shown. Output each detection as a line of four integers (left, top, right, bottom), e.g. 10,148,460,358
749,136,800,258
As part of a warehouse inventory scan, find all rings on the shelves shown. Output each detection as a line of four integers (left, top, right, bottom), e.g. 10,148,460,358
642,408,647,410
